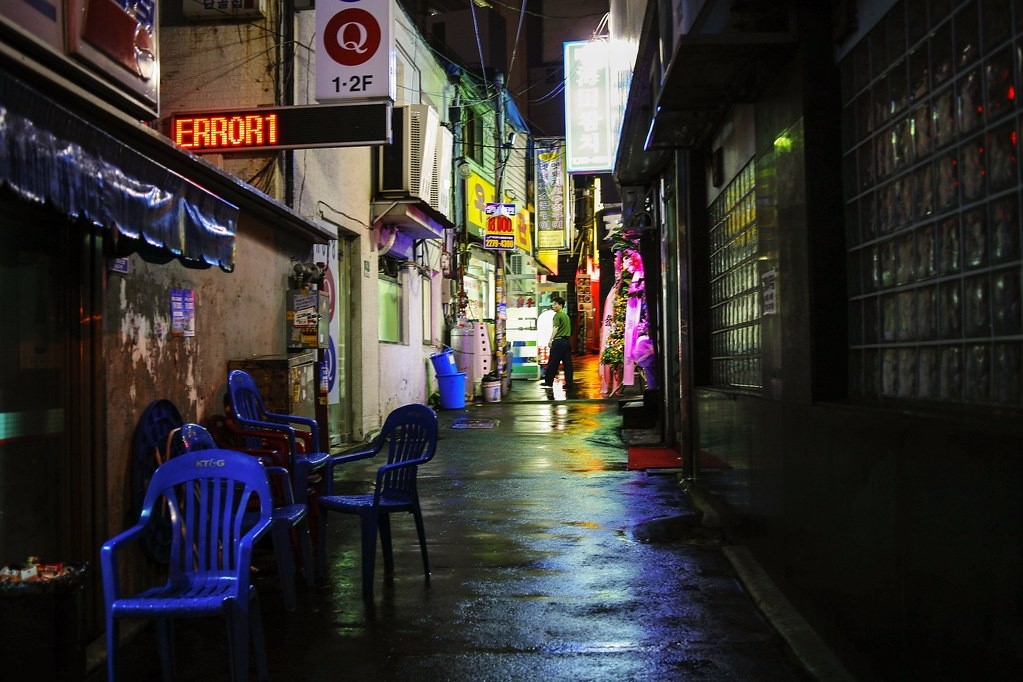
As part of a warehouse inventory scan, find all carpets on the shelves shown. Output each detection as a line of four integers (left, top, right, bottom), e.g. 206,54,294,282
698,450,731,467
626,447,680,470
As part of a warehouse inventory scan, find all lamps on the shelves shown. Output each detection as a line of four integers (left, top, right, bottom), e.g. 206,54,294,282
451,155,472,180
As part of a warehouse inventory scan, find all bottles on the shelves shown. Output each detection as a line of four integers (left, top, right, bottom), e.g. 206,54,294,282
25,555,41,568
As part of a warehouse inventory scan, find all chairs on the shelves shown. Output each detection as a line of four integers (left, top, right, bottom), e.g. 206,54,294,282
99,371,439,682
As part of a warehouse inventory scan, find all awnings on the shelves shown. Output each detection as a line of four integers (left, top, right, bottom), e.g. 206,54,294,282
0,70,243,272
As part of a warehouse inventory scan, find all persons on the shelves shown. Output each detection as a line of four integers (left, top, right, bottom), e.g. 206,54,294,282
539,296,574,389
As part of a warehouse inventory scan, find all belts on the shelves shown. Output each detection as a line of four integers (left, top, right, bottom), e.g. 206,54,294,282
554,336,570,340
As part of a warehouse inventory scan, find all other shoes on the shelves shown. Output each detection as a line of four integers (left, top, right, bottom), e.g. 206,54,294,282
541,382,552,387
563,384,574,389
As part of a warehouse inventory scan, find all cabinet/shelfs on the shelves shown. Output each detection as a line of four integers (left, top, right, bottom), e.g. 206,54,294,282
286,289,330,350
228,353,315,447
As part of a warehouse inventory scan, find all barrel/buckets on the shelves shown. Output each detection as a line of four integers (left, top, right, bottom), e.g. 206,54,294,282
429,348,458,374
480,380,502,403
435,372,466,410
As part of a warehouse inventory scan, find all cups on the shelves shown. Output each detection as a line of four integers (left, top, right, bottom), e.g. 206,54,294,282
312,283,317,290
